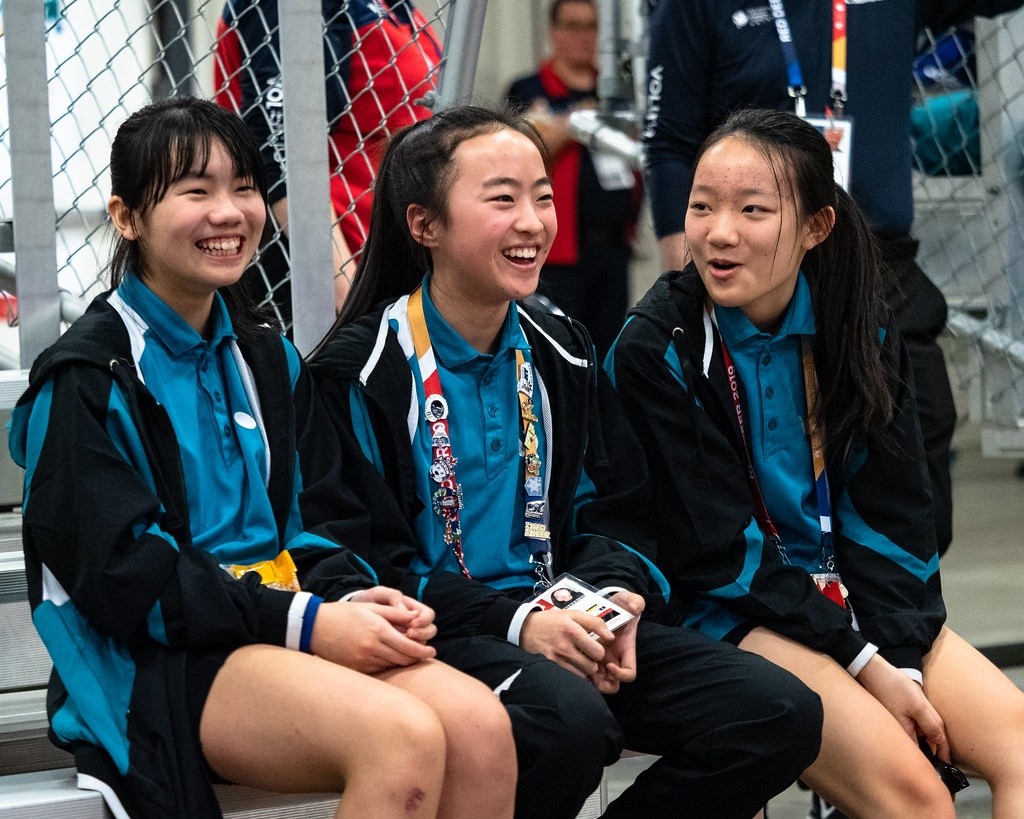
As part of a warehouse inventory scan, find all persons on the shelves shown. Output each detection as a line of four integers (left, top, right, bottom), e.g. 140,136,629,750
552,587,583,609
212,0,445,308
497,0,647,365
822,126,842,150
7,94,518,819
637,1,1023,557
292,102,825,819
568,108,1024,819
